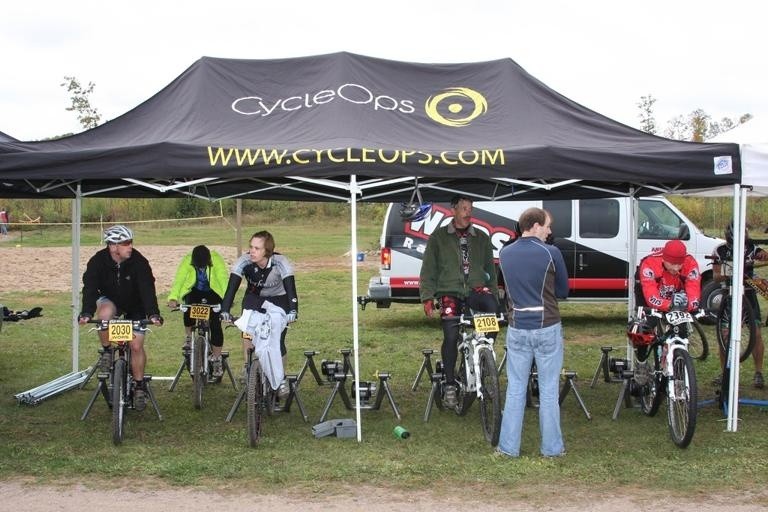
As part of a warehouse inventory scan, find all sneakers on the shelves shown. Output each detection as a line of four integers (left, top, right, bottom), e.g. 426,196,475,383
182,337,191,347
634,355,649,385
210,357,222,374
753,373,764,388
100,350,112,373
443,384,458,410
276,381,293,398
134,389,145,411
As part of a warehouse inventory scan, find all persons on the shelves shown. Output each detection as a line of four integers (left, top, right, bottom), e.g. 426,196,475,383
633,239,702,388
496,207,570,459
419,194,502,407
219,230,298,399
166,244,236,378
713,218,768,387
0,207,9,236
78,225,163,411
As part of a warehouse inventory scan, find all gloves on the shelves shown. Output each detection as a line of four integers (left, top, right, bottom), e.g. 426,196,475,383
424,302,436,318
671,290,689,309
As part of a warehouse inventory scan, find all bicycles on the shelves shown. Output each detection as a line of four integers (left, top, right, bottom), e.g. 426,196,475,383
165,298,236,411
655,301,709,362
629,306,721,449
82,310,161,446
704,254,763,364
218,309,298,450
441,299,512,446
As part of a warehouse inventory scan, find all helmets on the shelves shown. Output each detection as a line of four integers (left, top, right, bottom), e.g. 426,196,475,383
725,220,747,242
104,225,134,243
400,203,432,220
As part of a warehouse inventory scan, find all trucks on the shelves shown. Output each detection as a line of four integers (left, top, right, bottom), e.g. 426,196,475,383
356,195,728,328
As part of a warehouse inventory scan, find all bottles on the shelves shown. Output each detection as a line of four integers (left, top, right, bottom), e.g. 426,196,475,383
392,424,411,440
656,343,668,367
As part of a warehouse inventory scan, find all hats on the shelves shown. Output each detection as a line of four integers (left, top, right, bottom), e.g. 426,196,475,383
663,238,687,263
